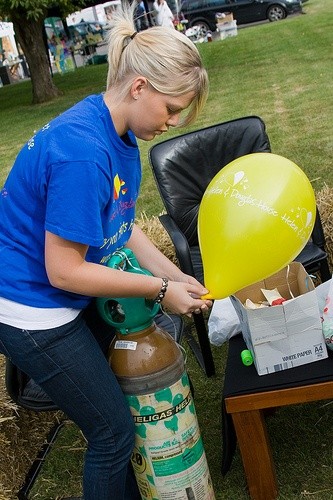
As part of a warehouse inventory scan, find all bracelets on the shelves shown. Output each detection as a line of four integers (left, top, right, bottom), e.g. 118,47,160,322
153,278,168,304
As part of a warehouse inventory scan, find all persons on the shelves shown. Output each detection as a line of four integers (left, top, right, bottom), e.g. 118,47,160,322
0,3,209,500
151,0,176,30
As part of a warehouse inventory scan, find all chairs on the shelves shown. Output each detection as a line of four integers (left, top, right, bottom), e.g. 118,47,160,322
149,116,330,378
5,303,194,500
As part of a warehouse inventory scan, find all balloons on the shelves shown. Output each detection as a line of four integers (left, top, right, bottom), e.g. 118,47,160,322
197,153,316,301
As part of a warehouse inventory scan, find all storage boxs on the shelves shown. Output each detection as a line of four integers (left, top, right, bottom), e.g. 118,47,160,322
215,13,238,40
228,262,329,378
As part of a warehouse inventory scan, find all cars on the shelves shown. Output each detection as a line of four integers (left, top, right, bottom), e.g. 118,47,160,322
177,0,304,39
57,21,104,56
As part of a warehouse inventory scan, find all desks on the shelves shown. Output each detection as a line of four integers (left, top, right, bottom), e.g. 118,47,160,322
224,331,333,500
